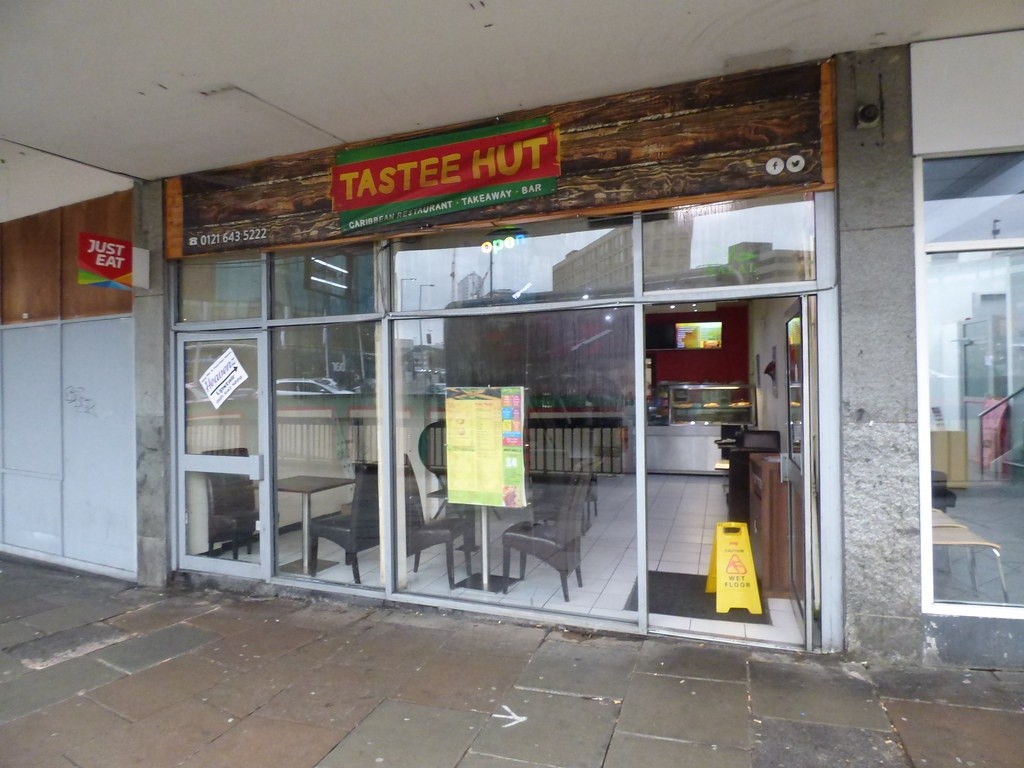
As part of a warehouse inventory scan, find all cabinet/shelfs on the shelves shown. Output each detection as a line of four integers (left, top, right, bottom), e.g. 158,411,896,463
671,382,757,427
750,450,814,601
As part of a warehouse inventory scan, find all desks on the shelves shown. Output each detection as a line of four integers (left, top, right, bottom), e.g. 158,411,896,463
244,475,356,574
715,459,729,470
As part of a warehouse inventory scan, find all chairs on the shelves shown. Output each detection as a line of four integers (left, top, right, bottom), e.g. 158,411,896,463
720,423,782,527
930,469,1011,602
405,419,599,601
200,447,281,561
310,463,379,586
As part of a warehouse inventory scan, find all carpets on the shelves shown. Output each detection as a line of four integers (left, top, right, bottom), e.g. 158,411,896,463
624,570,775,626
